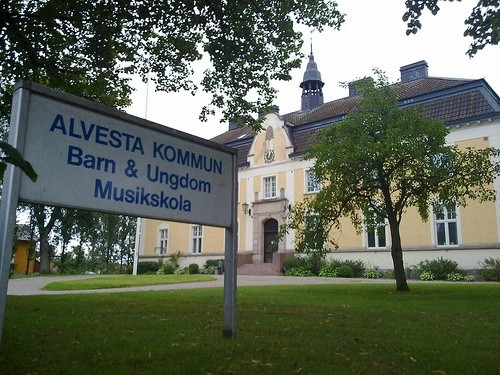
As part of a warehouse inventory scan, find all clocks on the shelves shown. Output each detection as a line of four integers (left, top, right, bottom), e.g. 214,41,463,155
264,149,275,163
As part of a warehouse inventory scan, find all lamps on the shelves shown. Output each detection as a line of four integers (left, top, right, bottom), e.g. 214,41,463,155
241,202,252,217
281,196,292,214
